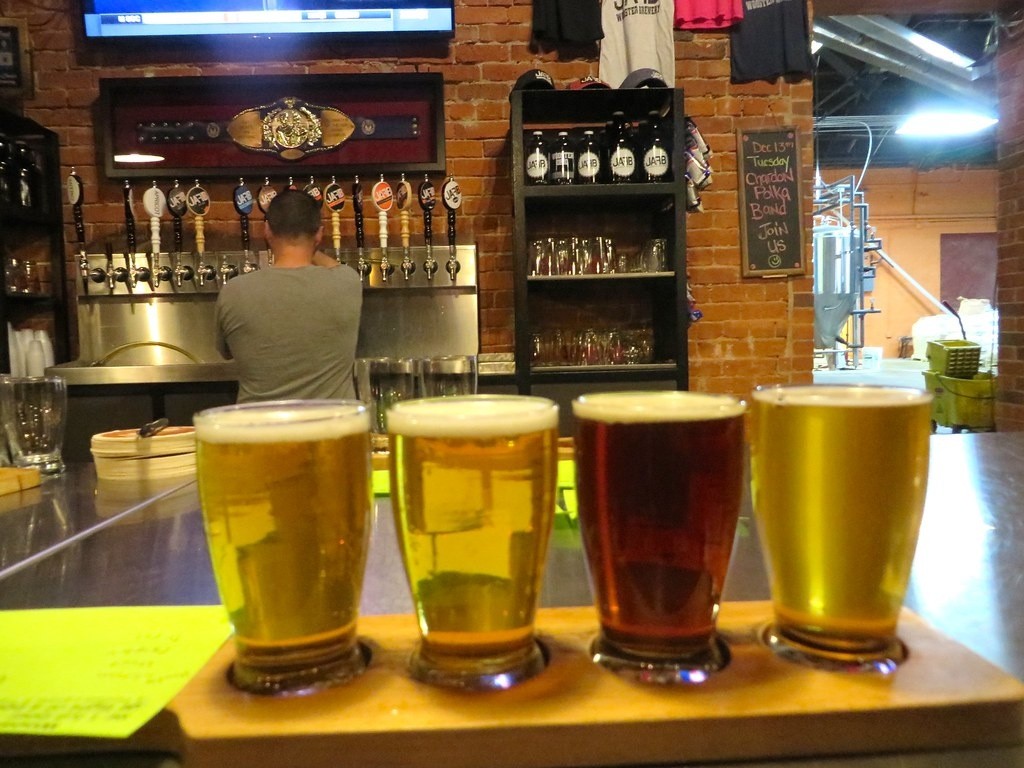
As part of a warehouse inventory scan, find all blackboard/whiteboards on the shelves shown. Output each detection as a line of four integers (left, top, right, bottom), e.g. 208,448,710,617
734,123,807,280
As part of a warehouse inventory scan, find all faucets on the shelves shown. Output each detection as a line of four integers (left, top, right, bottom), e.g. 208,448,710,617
66,255,463,284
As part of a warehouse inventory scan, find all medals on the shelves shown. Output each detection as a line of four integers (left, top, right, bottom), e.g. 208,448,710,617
225,96,357,162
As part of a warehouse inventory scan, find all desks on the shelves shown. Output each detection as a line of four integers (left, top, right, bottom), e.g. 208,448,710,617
0,433,1024,768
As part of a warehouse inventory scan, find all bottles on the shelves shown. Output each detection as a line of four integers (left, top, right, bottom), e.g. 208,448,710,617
528,104,669,184
0,129,46,208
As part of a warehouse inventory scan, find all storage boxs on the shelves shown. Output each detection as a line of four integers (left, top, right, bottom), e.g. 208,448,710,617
925,337,981,380
921,368,996,435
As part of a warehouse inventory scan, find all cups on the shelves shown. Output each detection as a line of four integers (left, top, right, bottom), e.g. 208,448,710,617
2,255,40,294
384,384,558,690
1,370,71,476
571,384,749,686
751,369,935,676
193,397,376,693
528,323,658,364
528,232,670,275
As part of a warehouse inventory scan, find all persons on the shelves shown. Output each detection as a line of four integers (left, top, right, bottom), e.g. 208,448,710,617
213,189,364,406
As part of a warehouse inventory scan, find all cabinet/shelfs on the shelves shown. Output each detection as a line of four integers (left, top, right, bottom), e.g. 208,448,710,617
0,113,72,380
509,86,689,441
